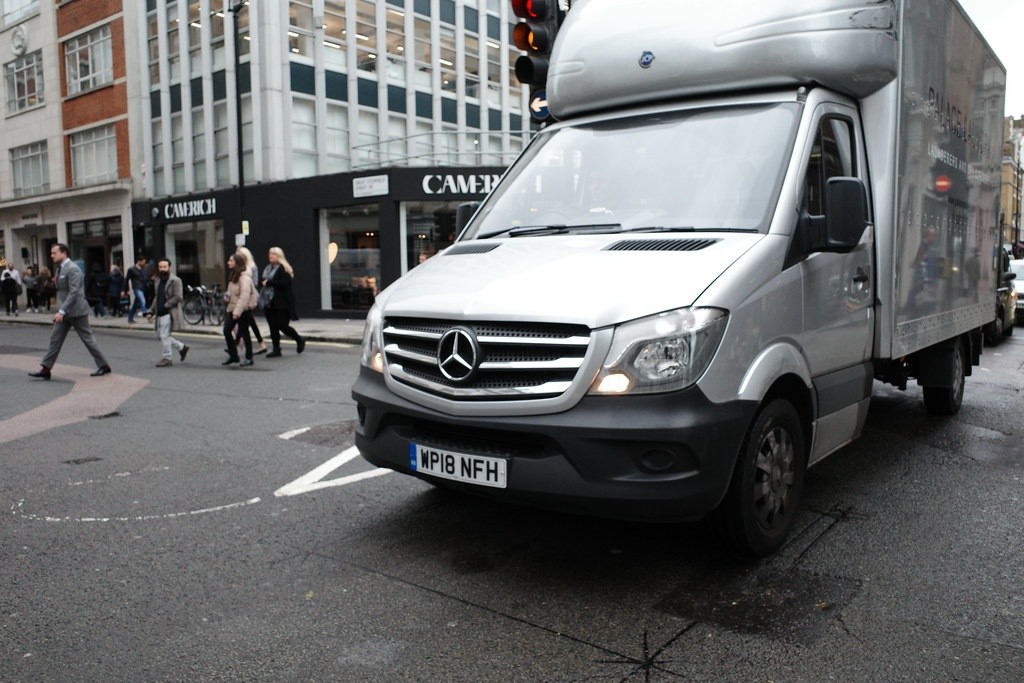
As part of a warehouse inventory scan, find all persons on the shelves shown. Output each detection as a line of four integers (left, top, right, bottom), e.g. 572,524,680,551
28,243,111,379
0,263,54,315
146,258,189,367
222,245,307,367
85,256,154,325
420,251,431,265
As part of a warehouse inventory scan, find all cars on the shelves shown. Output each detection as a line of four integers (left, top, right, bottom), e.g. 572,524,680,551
983,241,1024,346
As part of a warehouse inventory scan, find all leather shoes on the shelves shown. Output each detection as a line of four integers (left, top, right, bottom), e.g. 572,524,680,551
28,372,51,380
90,366,111,376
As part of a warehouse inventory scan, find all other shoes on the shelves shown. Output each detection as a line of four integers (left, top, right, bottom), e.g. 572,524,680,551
240,359,253,367
266,351,281,357
254,348,266,354
5,306,50,317
179,345,190,362
224,349,229,353
129,320,136,323
155,359,173,367
222,356,240,365
148,315,156,322
296,339,305,353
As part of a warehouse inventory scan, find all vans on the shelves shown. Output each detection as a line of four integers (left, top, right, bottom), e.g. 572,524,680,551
348,0,1006,557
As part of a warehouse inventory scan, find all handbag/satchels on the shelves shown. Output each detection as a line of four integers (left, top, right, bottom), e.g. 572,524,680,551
240,275,260,309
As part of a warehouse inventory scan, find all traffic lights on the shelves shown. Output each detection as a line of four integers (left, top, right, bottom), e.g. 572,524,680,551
511,0,566,125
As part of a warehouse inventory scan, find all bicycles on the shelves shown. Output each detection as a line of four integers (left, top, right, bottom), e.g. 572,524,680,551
181,283,227,327
